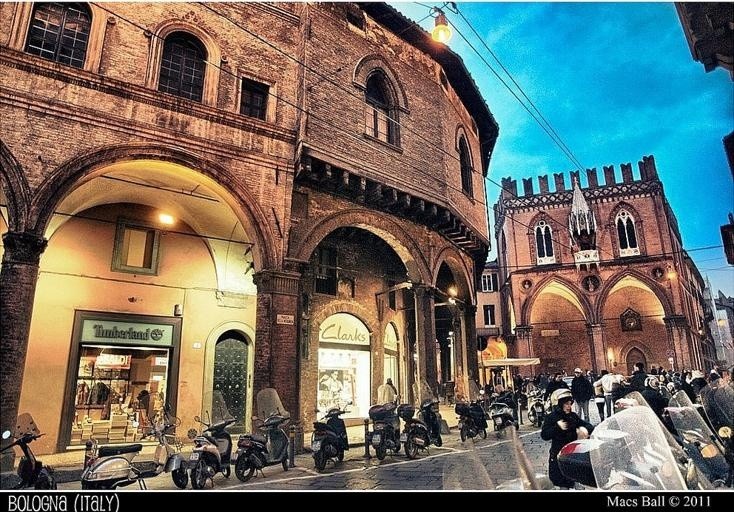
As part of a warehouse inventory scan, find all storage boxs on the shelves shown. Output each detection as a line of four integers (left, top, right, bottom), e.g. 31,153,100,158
70,414,143,446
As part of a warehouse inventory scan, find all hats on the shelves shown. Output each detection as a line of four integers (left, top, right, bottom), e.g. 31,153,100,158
574,367,582,373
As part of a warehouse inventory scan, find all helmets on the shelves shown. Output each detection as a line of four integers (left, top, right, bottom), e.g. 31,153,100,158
551,388,573,407
644,375,660,390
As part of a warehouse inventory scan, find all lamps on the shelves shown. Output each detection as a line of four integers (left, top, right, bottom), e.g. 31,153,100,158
432,9,453,43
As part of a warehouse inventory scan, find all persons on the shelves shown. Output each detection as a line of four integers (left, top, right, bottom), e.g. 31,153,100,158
517,362,734,430
540,388,594,490
319,370,352,405
386,377,401,399
